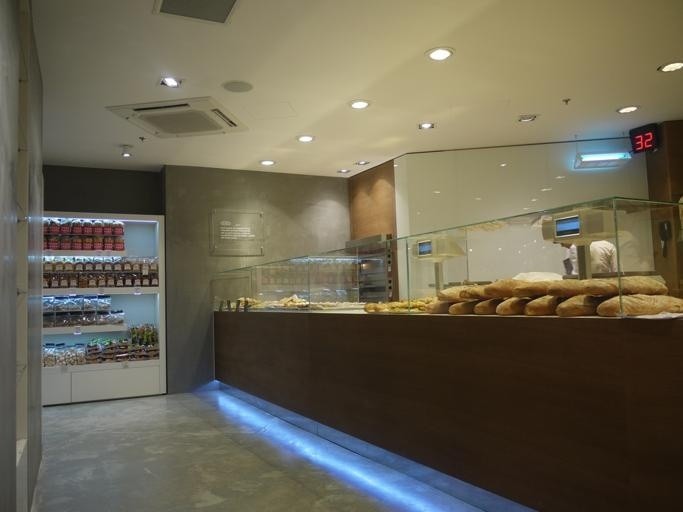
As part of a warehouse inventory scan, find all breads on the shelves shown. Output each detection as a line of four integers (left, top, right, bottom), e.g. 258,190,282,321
363,274,683,320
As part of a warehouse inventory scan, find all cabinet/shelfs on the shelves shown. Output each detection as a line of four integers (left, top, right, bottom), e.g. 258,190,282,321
41,211,170,406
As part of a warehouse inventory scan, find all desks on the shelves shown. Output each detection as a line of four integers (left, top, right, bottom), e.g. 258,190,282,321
213,311,683,509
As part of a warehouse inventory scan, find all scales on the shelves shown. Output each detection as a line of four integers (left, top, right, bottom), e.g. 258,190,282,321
542,208,657,280
412,236,493,296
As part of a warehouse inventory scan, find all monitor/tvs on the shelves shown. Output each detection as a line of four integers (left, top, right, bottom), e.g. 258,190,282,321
556,215,579,236
419,240,432,255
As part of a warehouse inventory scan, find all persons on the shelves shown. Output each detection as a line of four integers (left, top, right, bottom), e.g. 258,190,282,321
552,240,618,275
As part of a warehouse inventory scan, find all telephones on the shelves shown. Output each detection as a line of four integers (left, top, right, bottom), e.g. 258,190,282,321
659,222,671,241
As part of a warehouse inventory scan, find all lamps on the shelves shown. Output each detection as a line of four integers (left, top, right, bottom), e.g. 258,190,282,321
574,151,631,170
